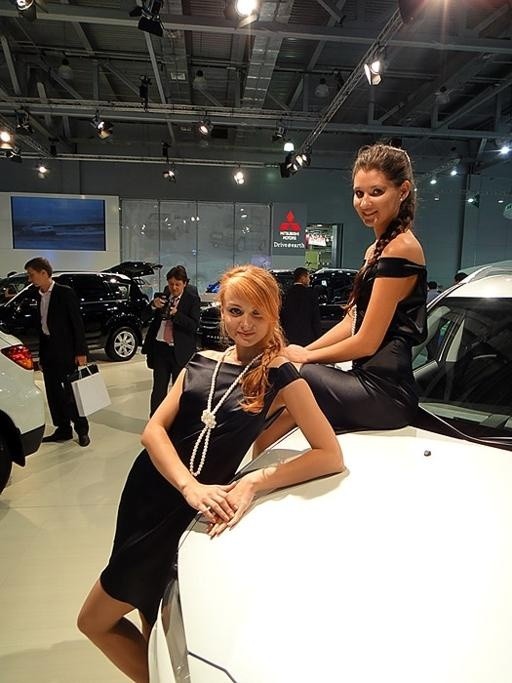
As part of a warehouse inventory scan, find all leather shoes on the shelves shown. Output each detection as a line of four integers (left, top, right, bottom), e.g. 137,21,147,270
43,432,73,442
79,435,90,446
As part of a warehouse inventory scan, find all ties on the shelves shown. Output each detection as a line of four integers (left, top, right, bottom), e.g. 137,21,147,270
164,296,179,343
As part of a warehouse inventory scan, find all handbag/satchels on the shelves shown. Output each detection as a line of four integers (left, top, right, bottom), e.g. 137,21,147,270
61,364,111,417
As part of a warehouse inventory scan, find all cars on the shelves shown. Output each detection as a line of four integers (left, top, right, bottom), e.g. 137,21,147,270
143,256,512,678
0,260,158,360
1,323,47,496
197,278,220,305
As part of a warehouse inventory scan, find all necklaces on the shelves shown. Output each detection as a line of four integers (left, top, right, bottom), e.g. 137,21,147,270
350,241,375,336
189,345,264,475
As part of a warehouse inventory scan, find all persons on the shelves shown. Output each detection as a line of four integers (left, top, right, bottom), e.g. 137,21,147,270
425,281,441,303
78,266,346,683
281,266,322,346
24,257,91,445
141,265,199,418
454,271,468,284
251,142,429,463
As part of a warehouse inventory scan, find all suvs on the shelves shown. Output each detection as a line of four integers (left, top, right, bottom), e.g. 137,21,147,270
196,268,360,352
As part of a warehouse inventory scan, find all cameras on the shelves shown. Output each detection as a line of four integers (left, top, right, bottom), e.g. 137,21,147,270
159,301,175,321
